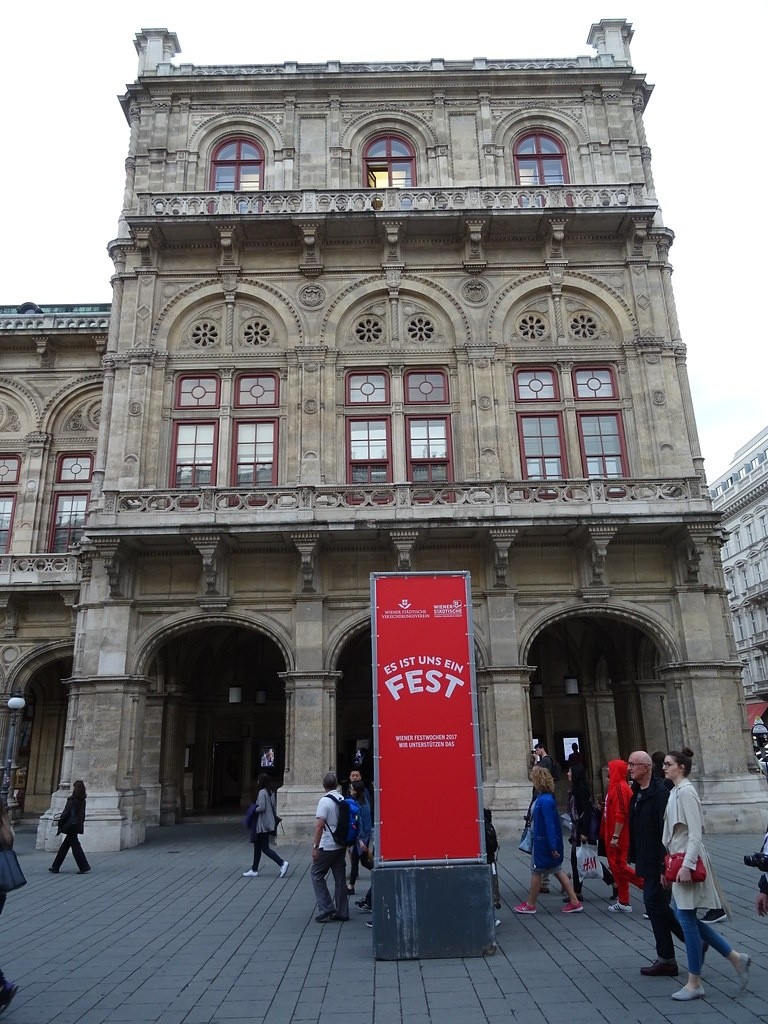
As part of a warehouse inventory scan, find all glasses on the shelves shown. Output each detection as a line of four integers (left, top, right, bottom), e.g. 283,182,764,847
628,762,643,768
662,760,681,768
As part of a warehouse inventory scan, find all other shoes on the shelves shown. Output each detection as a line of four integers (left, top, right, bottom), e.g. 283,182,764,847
311,883,372,927
0,849,27,894
739,953,752,991
77,866,91,874
0,983,19,1017
672,983,706,1000
494,874,650,925
48,868,59,873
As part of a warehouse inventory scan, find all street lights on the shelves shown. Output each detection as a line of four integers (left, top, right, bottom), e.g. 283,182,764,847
0,687,25,810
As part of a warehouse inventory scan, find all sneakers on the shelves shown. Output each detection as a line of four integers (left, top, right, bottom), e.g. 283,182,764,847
701,907,727,924
243,869,259,877
280,861,289,878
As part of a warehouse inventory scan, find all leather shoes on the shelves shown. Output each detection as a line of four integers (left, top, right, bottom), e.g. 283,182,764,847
701,940,709,965
640,958,677,976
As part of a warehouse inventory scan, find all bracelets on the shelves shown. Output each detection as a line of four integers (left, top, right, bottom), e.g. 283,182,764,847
312,843,319,849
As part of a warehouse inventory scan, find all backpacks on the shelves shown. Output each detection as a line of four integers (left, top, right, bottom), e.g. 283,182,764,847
484,821,497,853
546,756,561,782
325,794,363,846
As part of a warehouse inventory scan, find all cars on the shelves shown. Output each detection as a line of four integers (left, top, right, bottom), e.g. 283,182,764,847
756,751,768,774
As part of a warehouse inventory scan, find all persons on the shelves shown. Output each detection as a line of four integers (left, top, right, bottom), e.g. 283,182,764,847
662,746,752,1001
311,775,351,923
351,770,376,927
519,743,729,924
513,766,584,914
744,827,768,917
484,808,502,928
48,780,92,873
0,794,27,1014
242,773,289,877
627,751,709,975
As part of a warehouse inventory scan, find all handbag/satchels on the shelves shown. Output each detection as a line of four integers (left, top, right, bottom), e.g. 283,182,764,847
665,852,706,881
575,838,604,883
61,800,79,834
270,815,282,837
519,826,533,855
